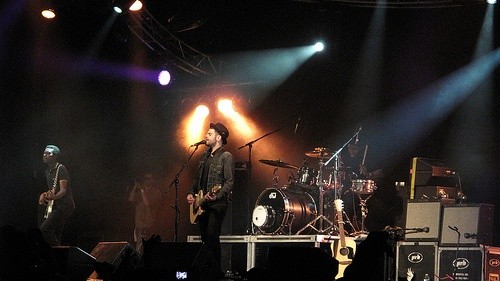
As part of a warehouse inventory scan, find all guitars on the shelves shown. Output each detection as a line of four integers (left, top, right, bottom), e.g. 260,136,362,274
330,199,357,279
189,184,222,224
38,186,57,228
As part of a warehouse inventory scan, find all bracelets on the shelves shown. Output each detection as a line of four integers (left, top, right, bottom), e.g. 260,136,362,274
141,189,144,192
45,192,47,197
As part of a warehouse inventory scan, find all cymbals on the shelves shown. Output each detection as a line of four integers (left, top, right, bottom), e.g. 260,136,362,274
258,159,299,169
304,147,334,159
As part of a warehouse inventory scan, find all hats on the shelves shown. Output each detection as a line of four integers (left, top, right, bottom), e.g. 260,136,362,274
209,122,229,145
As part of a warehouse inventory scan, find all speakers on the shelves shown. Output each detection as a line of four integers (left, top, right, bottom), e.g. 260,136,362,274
439,203,481,247
405,199,440,242
49,245,97,281
219,169,250,235
89,241,141,281
264,245,339,281
137,241,225,281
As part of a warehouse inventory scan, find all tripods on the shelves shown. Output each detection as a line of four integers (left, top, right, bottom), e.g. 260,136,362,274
294,157,348,235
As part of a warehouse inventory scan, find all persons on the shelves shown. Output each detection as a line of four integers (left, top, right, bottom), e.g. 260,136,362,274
337,140,382,231
186,123,234,281
93,228,394,281
128,172,162,254
39,145,75,247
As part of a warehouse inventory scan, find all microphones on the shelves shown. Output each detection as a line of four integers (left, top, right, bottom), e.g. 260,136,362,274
463,232,488,240
416,227,429,232
191,140,206,147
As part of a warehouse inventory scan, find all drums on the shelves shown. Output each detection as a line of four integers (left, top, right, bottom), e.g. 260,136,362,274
252,166,376,235
